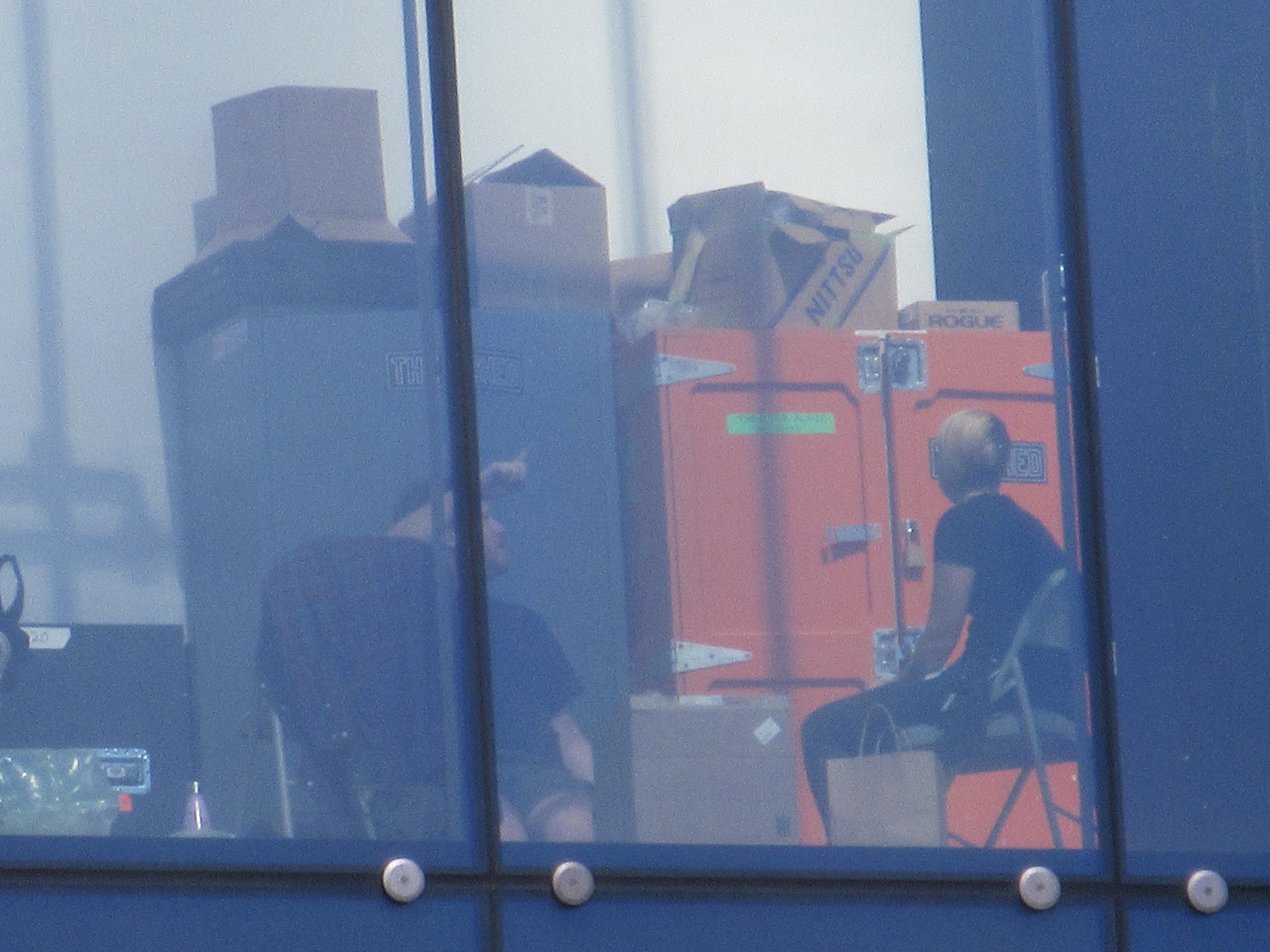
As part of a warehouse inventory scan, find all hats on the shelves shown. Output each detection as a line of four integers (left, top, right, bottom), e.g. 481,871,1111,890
445,518,509,575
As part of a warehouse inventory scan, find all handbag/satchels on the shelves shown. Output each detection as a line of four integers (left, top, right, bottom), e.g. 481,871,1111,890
827,704,948,848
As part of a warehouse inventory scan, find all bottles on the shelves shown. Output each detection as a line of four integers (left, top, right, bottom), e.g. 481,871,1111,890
184,780,210,829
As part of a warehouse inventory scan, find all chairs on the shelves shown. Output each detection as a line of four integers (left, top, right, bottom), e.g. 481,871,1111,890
256,540,471,840
941,568,1098,851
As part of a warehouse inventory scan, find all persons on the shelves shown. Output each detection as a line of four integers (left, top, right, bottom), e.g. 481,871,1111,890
387,442,598,840
801,408,1069,850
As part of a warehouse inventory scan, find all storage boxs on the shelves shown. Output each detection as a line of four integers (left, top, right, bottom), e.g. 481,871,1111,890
610,255,679,318
631,692,797,842
903,302,1018,329
668,184,907,336
398,144,608,311
202,84,413,256
